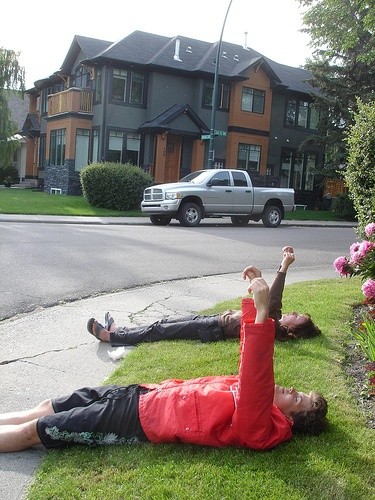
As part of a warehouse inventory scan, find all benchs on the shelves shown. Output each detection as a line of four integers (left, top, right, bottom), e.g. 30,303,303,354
294,205,307,210
51,188,61,194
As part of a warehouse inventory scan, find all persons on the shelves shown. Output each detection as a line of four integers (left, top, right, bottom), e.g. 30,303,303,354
87,246,321,348
0,264,329,452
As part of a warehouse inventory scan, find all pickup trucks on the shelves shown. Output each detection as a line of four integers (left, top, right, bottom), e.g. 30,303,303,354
140,169,296,227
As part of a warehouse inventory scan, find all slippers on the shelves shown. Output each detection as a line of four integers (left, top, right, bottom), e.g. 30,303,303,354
87,318,105,342
104,311,113,331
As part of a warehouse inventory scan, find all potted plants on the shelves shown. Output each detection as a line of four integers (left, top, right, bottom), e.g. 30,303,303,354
2,176,13,187
